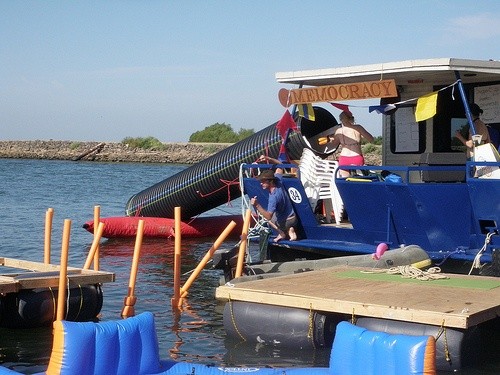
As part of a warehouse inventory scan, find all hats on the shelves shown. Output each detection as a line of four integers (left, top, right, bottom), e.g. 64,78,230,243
256,170,277,180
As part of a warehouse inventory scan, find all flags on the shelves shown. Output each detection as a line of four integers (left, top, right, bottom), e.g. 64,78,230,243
277,109,298,139
298,102,315,122
277,144,292,174
331,103,350,114
369,104,397,114
415,90,438,123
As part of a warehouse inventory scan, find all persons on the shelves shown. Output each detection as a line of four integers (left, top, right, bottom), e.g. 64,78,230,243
453,102,491,177
330,111,375,180
249,154,300,244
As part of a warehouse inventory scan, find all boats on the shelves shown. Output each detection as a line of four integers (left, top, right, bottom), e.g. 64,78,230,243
233,58,499,278
